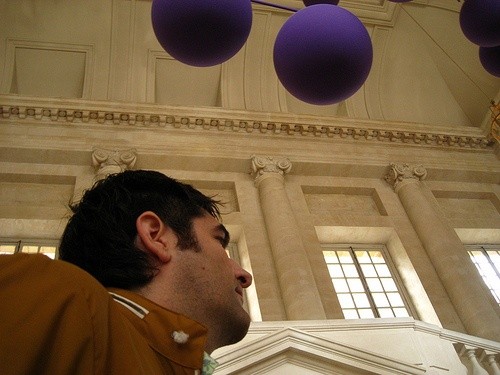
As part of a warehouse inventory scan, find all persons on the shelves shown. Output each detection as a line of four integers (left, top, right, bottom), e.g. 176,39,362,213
0,169,253,374
